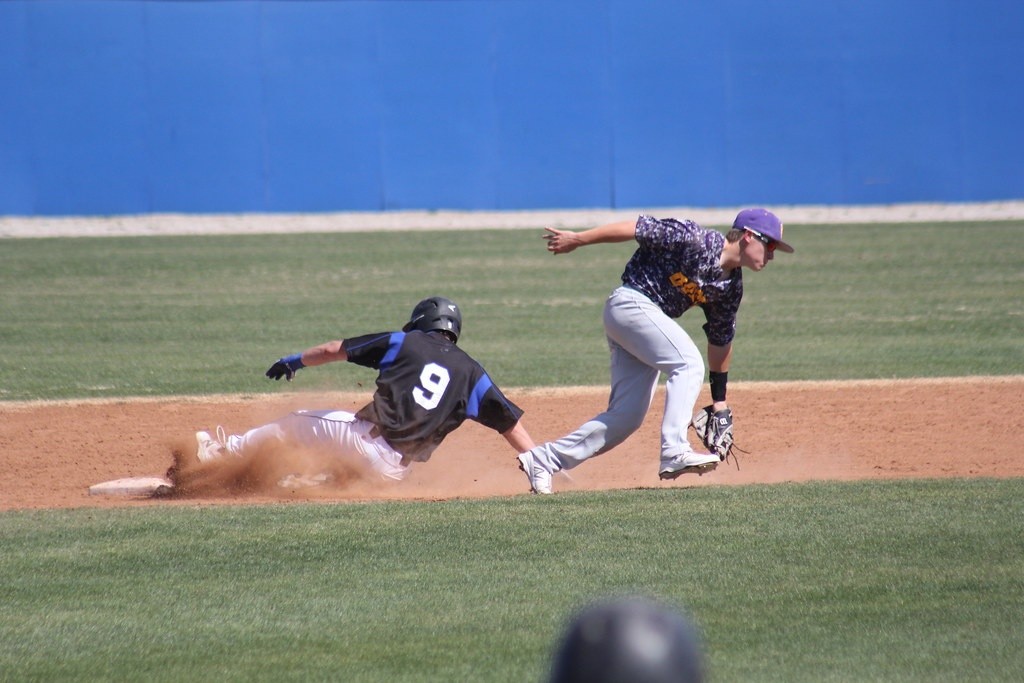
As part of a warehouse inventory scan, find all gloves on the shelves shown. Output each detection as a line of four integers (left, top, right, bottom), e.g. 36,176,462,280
265,352,306,382
560,469,573,480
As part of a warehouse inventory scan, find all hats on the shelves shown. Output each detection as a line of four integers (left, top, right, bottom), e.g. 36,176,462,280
731,209,794,254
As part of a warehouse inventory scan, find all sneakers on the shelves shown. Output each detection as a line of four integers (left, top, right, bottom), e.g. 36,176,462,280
195,430,223,465
515,450,552,496
659,452,721,480
276,466,340,491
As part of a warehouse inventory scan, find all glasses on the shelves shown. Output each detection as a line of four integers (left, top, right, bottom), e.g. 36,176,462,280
739,229,777,253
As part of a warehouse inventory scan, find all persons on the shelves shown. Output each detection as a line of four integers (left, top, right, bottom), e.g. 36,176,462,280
517,210,793,493
551,601,702,683
195,296,536,489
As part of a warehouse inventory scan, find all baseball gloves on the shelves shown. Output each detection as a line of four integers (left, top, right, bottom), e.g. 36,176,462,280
688,404,752,471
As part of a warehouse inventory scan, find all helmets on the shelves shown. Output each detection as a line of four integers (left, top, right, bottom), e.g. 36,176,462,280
402,297,462,345
553,597,703,682
